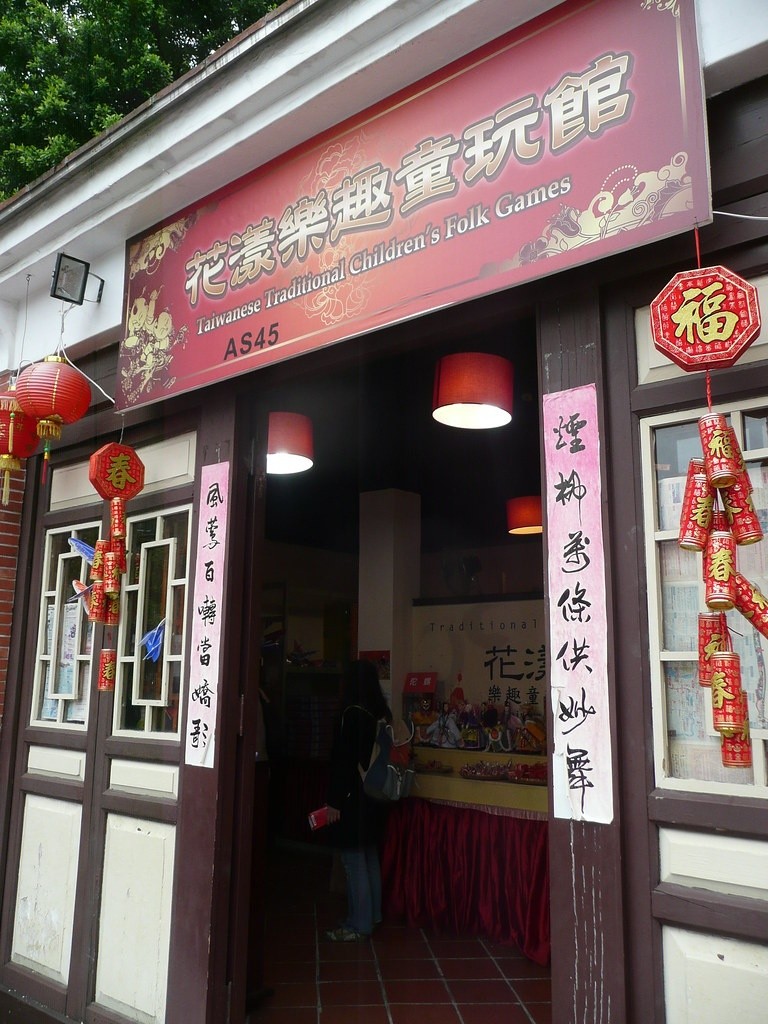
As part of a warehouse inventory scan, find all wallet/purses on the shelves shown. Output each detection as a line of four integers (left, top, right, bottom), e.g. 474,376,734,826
308,806,329,830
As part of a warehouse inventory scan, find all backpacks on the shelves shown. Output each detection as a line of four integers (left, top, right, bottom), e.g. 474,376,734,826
344,704,414,801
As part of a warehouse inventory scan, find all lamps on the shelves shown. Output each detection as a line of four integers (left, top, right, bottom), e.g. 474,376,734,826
250,411,316,476
50,252,105,306
432,354,515,432
506,494,546,537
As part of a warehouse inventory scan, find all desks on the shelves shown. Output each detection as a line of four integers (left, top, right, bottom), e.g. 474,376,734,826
379,745,551,967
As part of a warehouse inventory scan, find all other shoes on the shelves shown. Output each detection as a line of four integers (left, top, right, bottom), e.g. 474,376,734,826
317,921,378,945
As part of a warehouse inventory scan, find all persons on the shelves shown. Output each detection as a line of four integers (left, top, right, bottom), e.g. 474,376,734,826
322,658,392,941
